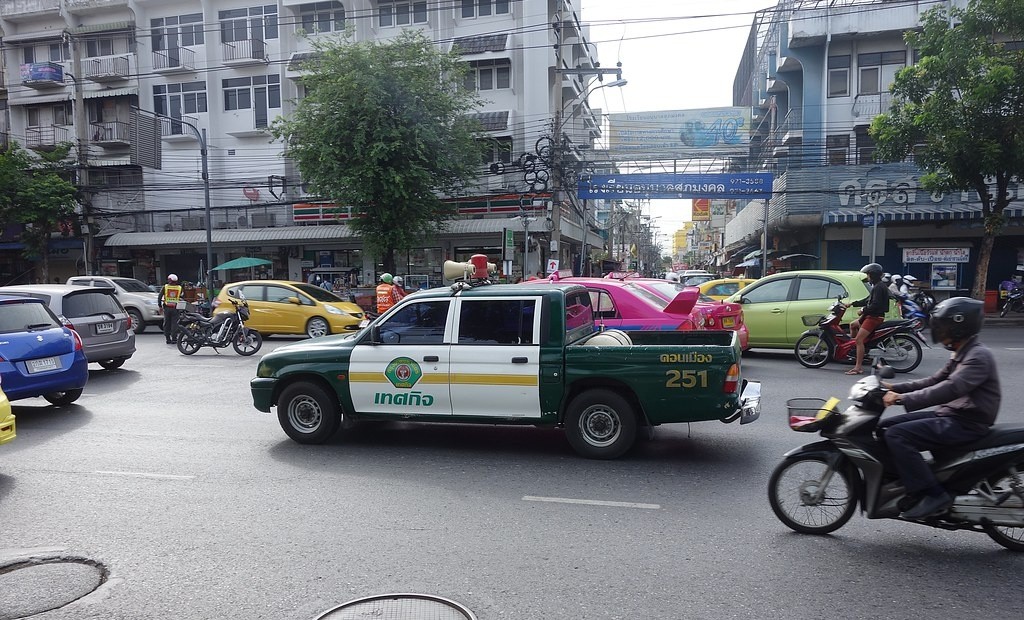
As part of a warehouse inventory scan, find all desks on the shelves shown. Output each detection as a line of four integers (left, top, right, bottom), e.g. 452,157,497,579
984,290,998,313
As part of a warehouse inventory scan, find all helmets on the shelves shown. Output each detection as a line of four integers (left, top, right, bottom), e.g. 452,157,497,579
392,276,404,287
881,272,892,283
928,296,985,345
890,273,903,284
903,275,918,287
380,272,393,284
860,263,883,285
167,274,178,282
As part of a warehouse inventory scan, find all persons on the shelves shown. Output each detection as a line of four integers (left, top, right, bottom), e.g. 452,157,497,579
665,268,679,281
536,270,544,279
514,276,523,284
681,121,706,146
770,266,776,272
158,274,182,344
308,272,315,284
844,263,890,375
320,278,333,293
35,277,40,284
883,273,918,300
376,273,407,315
54,277,60,283
1012,265,1024,283
876,296,1000,520
201,280,222,308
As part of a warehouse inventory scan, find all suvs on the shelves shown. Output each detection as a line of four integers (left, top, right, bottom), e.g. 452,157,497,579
0,283,137,369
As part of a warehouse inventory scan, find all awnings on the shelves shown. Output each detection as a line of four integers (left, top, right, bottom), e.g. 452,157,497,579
823,202,1024,224
105,224,364,246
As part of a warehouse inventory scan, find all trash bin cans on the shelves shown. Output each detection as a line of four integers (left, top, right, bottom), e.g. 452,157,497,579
985,290,998,313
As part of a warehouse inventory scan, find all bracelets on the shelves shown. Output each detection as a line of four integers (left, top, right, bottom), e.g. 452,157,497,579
861,309,863,312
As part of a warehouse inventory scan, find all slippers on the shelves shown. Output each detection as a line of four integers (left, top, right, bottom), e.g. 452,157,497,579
845,369,864,375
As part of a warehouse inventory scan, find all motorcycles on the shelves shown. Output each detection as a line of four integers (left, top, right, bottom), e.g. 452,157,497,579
177,288,262,355
794,291,933,372
766,361,1024,555
998,282,1024,319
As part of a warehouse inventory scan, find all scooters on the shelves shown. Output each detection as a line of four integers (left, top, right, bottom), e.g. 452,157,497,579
900,287,938,335
359,309,384,334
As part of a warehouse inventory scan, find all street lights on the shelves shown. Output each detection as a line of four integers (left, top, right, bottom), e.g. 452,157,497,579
548,74,629,279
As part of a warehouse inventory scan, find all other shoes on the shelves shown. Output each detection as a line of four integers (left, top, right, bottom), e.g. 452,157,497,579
166,339,171,344
171,340,177,344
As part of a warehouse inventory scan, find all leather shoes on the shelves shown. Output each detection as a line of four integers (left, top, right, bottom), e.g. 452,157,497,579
901,492,953,520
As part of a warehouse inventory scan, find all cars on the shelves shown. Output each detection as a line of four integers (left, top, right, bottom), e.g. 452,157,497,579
612,275,751,353
211,279,371,339
695,277,760,302
0,292,89,408
674,267,712,290
718,270,904,354
514,267,700,334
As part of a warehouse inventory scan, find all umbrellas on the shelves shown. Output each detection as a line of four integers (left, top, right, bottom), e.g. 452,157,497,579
213,256,273,281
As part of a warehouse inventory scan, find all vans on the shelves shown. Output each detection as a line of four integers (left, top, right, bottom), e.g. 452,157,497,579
66,276,168,336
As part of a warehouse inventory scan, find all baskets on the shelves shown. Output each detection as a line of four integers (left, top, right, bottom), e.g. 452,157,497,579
801,314,826,326
785,397,839,433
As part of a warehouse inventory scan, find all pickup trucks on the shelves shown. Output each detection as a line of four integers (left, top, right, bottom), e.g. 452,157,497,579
248,254,762,460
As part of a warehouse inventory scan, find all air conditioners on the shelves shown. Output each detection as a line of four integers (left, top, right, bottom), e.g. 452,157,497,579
163,217,183,232
487,161,504,177
236,215,252,229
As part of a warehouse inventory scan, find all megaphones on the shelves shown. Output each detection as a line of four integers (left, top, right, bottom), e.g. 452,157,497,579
468,259,497,274
444,260,476,280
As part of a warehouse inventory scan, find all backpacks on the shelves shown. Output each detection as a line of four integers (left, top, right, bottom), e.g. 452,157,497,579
322,282,329,291
